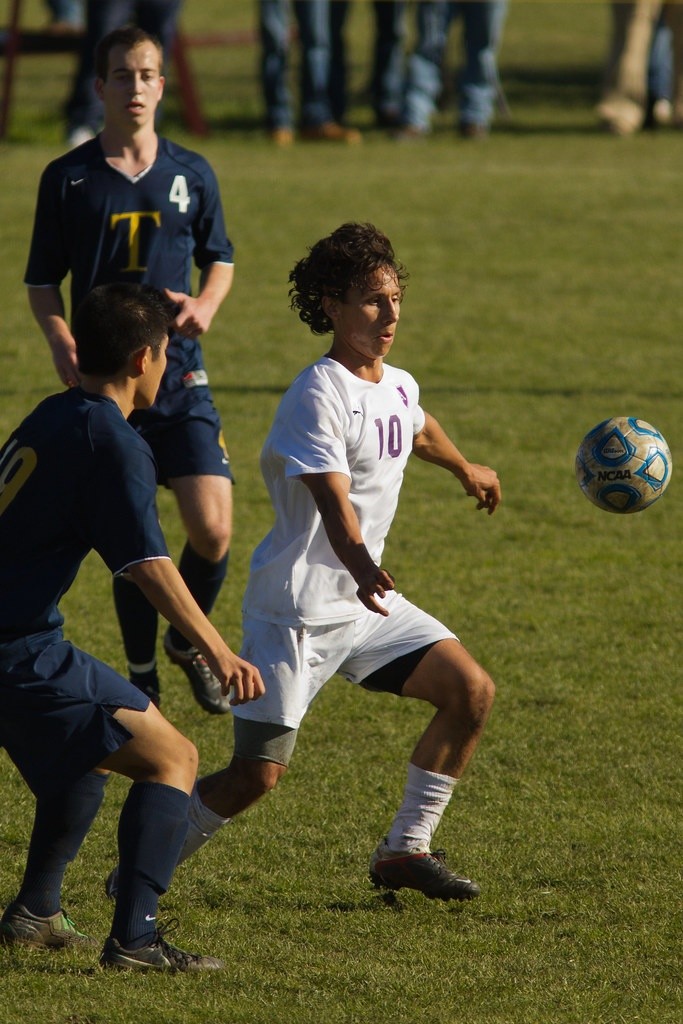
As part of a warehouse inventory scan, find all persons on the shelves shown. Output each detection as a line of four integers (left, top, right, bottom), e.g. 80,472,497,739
0,0,683,144
105,222,496,898
0,261,265,979
24,26,236,711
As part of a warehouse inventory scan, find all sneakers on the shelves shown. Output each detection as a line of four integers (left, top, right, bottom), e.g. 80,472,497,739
99,918,225,974
128,662,160,711
0,903,89,952
369,837,482,902
106,867,121,904
164,624,231,715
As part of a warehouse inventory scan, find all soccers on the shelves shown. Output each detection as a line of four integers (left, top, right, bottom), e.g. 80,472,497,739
574,417,673,515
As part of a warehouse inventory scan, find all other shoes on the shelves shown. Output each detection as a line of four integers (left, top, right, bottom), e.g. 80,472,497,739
406,123,428,138
378,105,404,126
70,124,96,148
461,121,489,138
308,120,361,142
272,125,295,146
